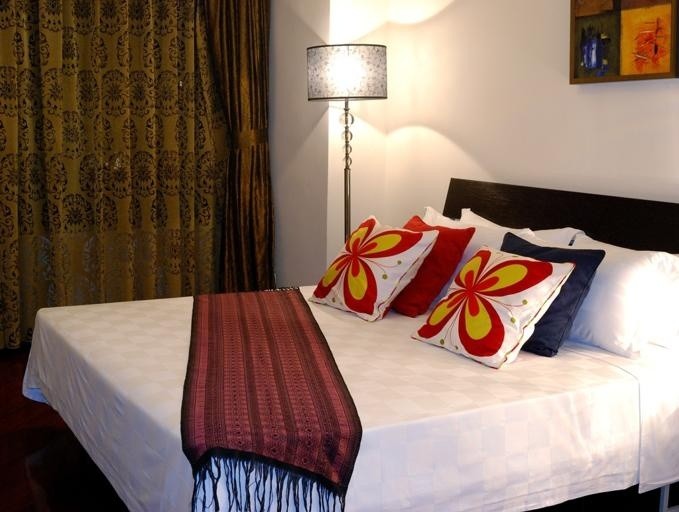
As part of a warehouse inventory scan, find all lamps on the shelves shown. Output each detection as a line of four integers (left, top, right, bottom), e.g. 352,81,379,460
306,44,387,244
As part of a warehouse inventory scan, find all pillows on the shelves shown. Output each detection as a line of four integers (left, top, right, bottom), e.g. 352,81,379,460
308,215,437,324
411,245,567,369
557,234,679,358
424,206,500,302
499,232,605,359
395,216,477,319
462,208,535,243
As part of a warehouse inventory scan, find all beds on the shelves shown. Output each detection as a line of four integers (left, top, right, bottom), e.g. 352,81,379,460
22,178,679,512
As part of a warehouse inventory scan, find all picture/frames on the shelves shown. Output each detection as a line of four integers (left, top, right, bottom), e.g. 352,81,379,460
569,0,679,84
